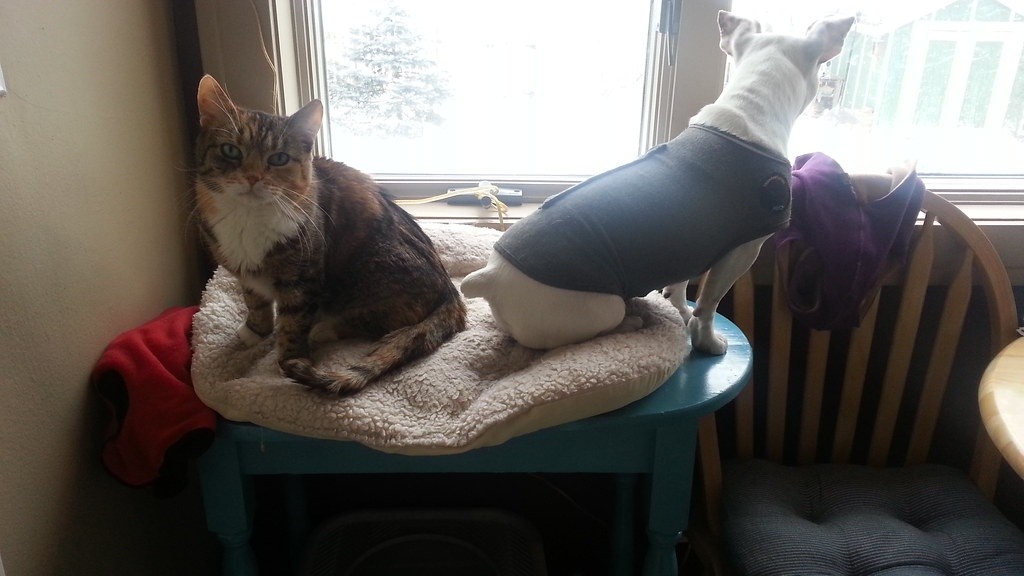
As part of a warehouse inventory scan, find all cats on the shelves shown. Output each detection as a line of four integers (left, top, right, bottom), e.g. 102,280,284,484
169,74,471,393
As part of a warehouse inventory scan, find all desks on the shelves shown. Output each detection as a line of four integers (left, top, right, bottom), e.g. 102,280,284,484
182,301,756,575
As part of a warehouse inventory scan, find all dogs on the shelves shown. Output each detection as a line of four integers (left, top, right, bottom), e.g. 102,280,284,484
457,7,857,358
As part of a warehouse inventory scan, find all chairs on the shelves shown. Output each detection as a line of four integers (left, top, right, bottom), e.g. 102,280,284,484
697,172,1024,576
